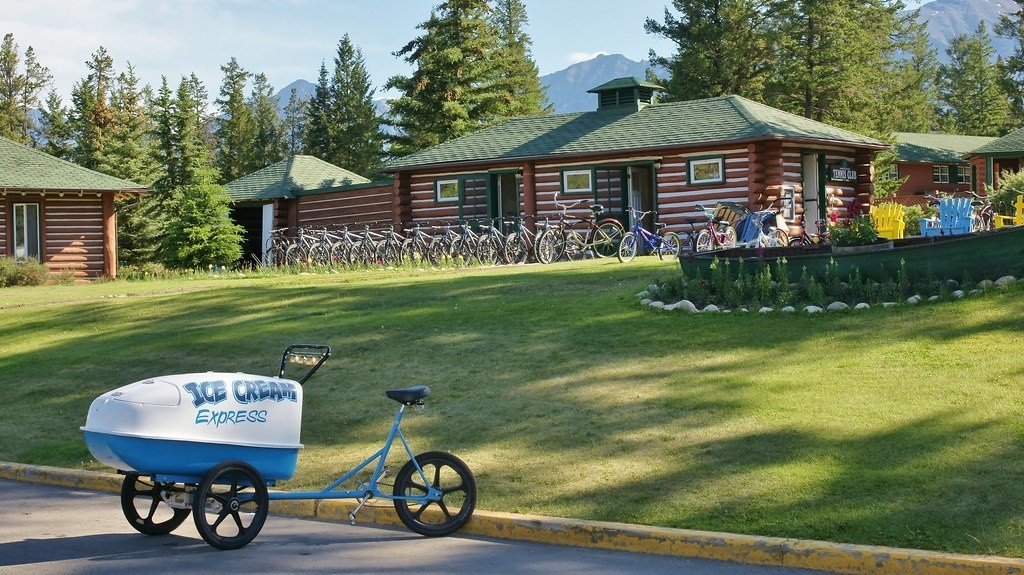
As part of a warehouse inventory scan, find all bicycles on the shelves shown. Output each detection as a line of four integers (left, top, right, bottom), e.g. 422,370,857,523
670,204,789,258
788,208,834,247
265,218,536,273
531,213,587,264
922,184,1024,233
476,216,527,267
618,204,681,264
504,214,557,265
537,189,625,264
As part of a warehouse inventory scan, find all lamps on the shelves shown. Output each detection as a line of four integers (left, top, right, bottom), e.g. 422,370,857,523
839,159,848,171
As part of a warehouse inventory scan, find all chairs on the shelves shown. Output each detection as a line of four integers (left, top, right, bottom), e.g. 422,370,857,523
868,202,906,239
917,197,975,238
993,195,1024,230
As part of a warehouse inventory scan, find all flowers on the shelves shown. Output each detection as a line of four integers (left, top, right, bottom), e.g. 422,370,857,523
827,198,876,244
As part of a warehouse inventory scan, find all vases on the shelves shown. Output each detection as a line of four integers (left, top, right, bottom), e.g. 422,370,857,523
829,240,894,254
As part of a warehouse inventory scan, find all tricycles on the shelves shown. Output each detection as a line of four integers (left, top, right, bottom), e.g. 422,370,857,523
79,344,478,551
